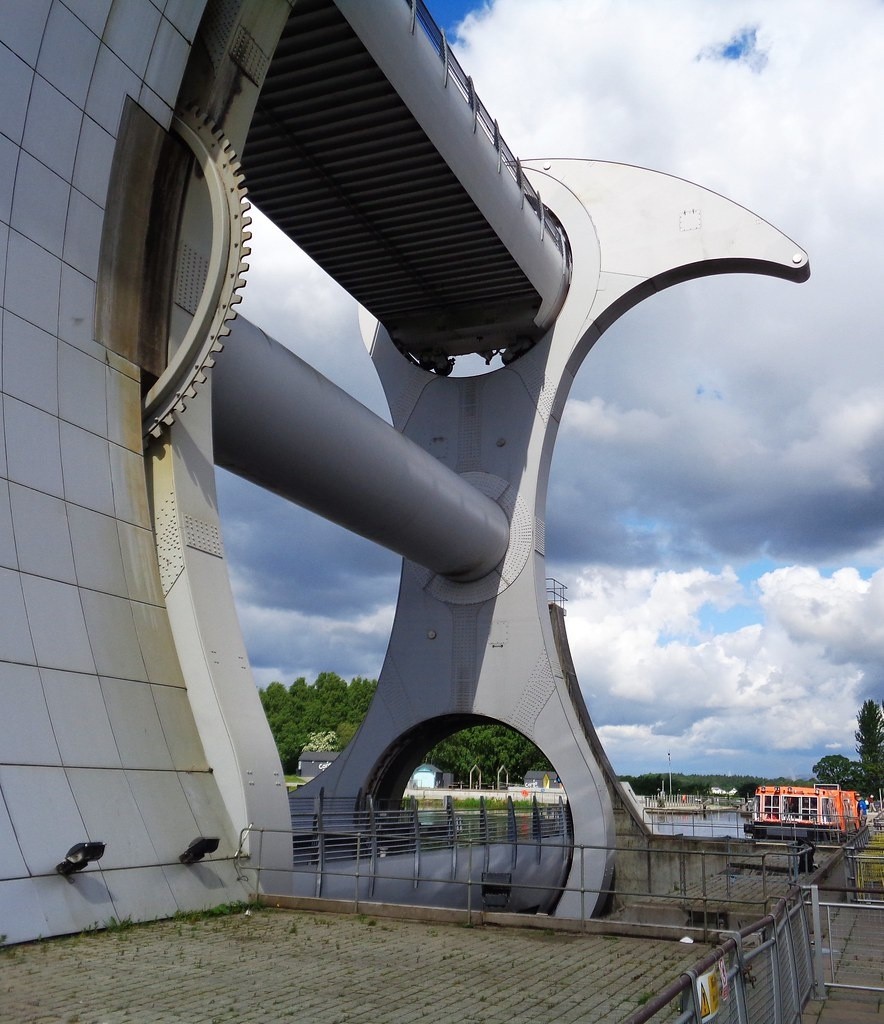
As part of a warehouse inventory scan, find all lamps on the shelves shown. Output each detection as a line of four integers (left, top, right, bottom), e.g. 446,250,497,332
56,841,107,883
178,836,221,867
480,871,512,911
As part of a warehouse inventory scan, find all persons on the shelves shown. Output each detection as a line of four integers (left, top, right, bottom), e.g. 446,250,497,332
864,796,870,813
855,793,867,827
869,795,877,812
